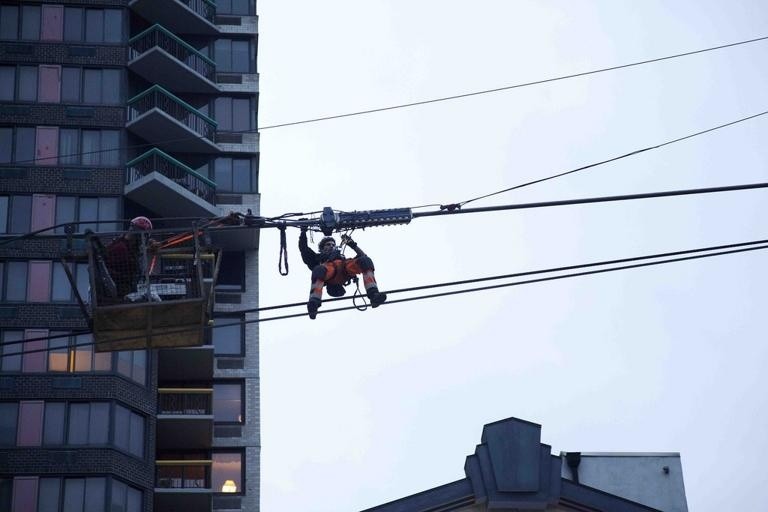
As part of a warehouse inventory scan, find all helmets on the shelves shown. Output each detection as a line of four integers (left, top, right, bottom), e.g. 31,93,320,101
130,216,153,236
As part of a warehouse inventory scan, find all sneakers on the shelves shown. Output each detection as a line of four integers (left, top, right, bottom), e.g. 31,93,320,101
369,292,387,309
307,301,318,320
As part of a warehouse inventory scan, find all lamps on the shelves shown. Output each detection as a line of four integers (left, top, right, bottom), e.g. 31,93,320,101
220,480,237,493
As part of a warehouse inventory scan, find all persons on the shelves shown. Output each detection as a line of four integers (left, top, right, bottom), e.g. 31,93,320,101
102,216,188,330
294,217,388,319
